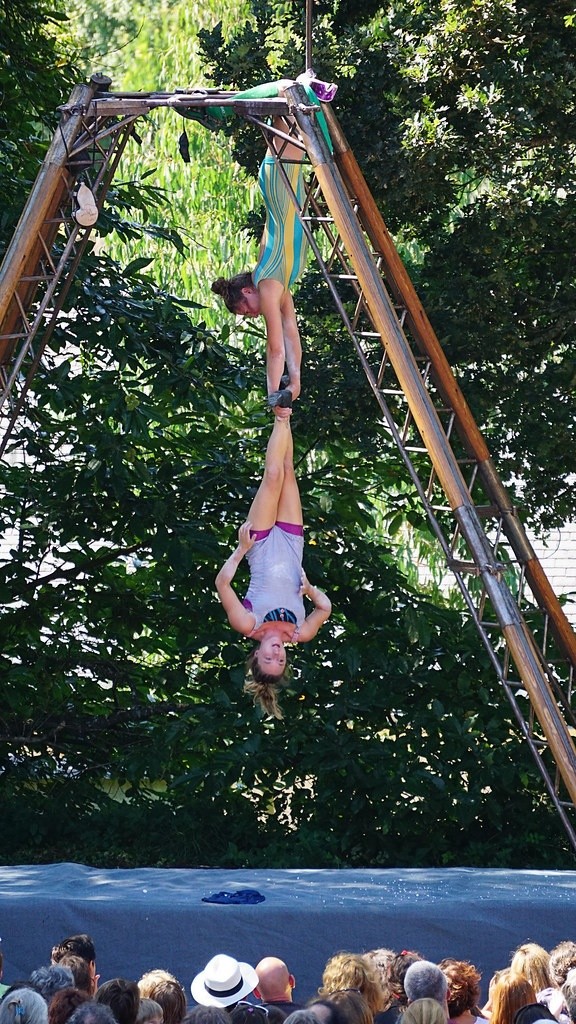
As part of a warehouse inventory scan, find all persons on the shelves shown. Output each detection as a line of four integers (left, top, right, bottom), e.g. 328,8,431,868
0,934,576,1024
173,80,308,421
214,389,331,719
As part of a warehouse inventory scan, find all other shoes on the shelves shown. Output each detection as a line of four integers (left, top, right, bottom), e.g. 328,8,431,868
270,390,292,408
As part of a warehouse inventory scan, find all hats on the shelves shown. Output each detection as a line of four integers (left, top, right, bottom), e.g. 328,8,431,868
191,954,259,1008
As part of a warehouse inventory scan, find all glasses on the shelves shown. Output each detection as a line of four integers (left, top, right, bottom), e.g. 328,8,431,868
329,989,362,995
233,1000,269,1019
